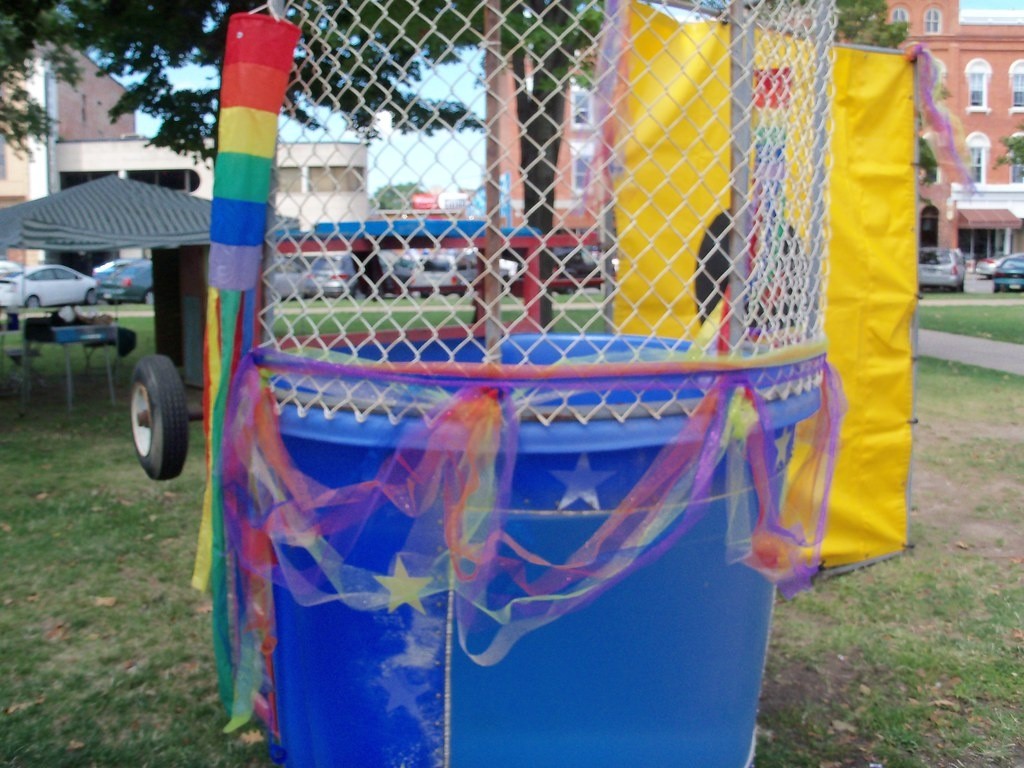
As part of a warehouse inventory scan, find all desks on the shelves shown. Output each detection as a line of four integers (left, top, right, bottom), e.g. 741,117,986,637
23,316,119,412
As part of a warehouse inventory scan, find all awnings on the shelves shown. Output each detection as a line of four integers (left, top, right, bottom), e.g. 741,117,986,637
957,209,1022,229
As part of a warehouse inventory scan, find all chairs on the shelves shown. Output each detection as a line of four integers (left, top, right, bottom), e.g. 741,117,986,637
1,324,42,389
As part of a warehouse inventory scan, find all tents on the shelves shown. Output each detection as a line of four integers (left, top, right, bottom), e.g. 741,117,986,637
1,173,302,417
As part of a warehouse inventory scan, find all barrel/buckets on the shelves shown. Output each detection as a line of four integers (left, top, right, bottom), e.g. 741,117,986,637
259,334,821,768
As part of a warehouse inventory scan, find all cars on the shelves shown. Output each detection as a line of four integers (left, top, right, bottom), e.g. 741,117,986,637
0,261,21,274
92,258,148,284
382,248,520,287
550,246,615,294
992,258,1024,292
918,248,965,292
977,253,1024,279
271,258,308,296
304,255,359,299
101,261,154,305
0,264,98,308
